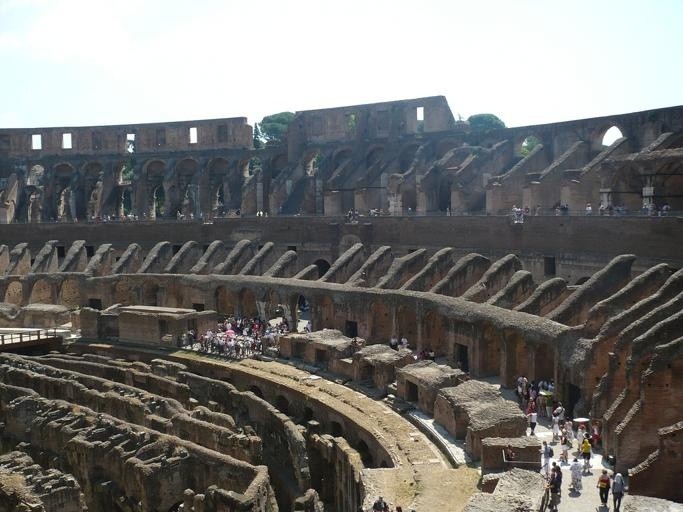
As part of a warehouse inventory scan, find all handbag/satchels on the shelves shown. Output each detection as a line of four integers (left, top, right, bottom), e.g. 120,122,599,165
600,482,607,489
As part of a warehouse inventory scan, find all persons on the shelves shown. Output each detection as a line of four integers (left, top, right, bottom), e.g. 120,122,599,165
176,210,180,220
446,205,450,216
504,444,514,461
180,316,312,358
85,212,138,222
256,208,262,216
372,496,389,511
516,374,623,512
345,207,380,223
389,335,434,361
511,201,671,221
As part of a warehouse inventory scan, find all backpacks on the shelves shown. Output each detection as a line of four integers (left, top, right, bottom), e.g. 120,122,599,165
549,447,554,457
